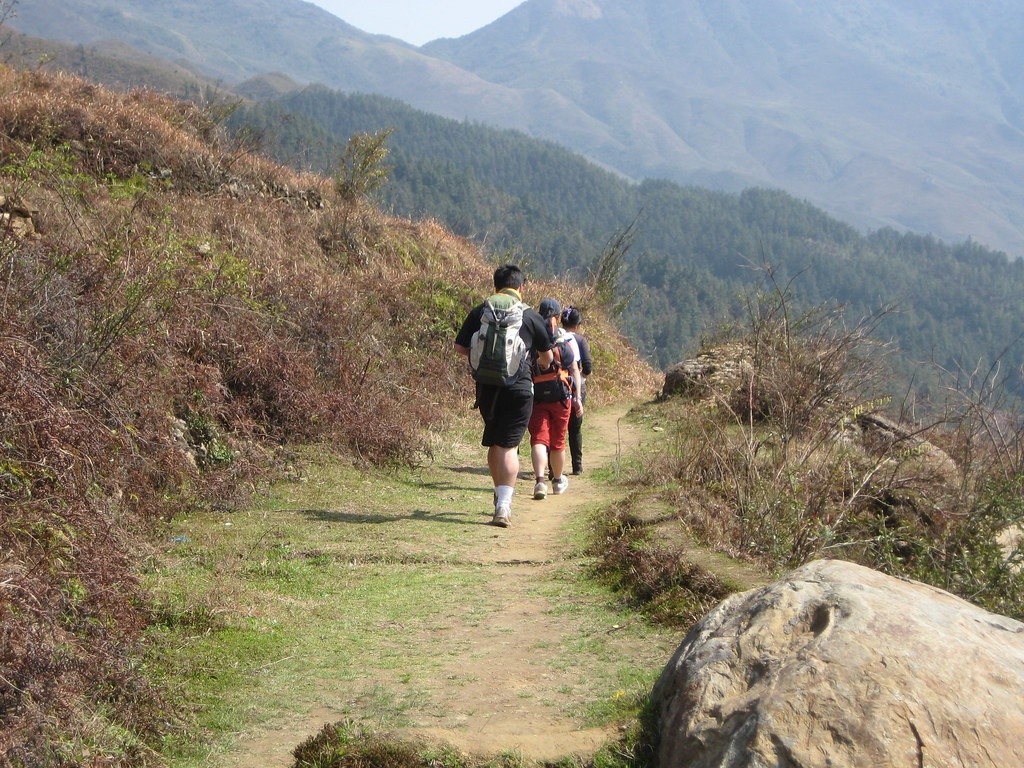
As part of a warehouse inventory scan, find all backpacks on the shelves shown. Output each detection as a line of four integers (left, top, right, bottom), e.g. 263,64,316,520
530,333,576,403
468,297,531,390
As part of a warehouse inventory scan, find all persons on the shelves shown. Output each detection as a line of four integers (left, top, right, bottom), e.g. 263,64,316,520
528,300,592,500
454,264,553,526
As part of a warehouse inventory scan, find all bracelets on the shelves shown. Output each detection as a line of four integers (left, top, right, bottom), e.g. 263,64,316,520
573,396,582,403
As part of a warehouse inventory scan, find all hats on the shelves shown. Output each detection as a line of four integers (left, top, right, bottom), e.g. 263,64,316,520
538,300,561,319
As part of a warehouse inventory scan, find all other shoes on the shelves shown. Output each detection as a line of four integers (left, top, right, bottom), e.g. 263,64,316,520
573,467,583,475
493,494,511,526
548,469,554,481
534,483,548,500
551,475,569,495
